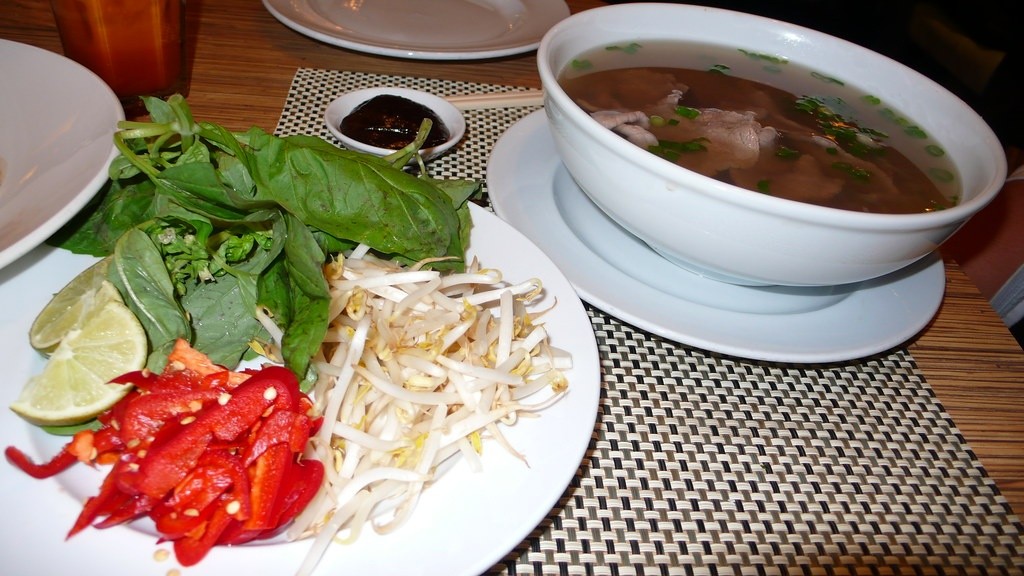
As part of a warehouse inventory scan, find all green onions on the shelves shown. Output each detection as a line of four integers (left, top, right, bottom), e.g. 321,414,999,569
570,44,959,214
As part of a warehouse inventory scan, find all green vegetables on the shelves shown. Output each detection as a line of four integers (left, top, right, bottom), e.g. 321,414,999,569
41,93,480,394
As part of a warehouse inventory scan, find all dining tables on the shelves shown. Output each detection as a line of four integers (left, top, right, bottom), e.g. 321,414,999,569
0,0,1024,576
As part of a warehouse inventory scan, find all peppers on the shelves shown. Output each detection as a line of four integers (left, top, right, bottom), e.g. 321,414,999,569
5,338,325,567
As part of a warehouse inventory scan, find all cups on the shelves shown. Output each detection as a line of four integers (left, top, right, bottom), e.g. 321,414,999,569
49,0,183,120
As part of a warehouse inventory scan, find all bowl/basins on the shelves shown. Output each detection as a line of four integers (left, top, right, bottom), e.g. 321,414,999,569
536,5,1011,291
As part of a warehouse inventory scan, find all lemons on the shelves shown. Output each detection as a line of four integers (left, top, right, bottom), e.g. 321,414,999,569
9,254,147,426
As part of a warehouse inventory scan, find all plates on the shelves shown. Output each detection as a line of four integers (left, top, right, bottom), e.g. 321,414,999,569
320,85,466,163
259,0,571,61
486,103,947,365
1,202,604,575
1,38,128,274
